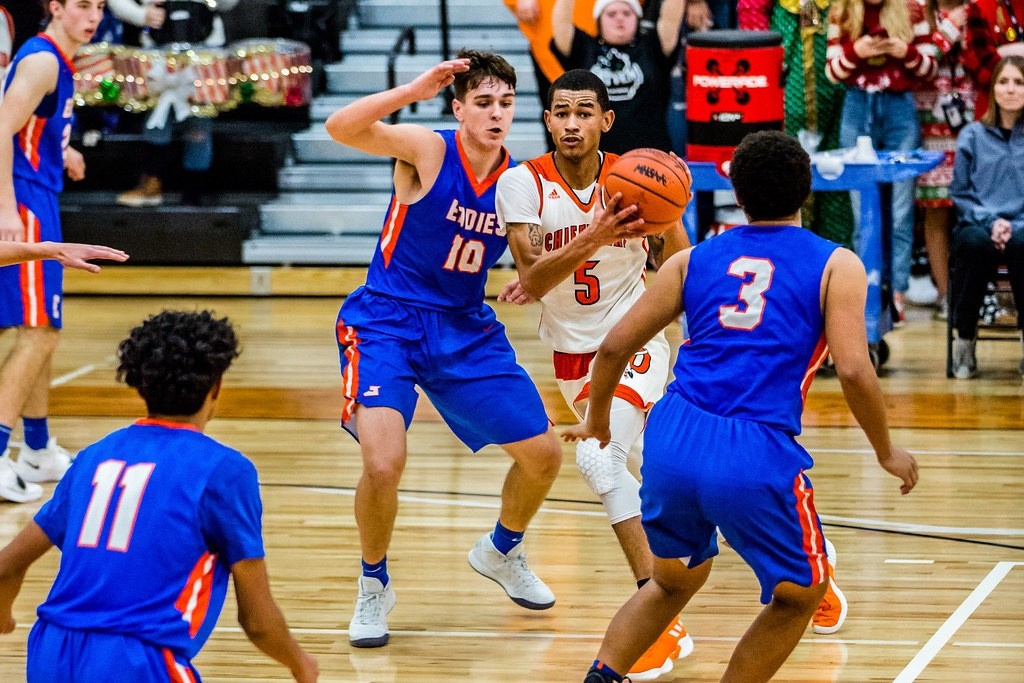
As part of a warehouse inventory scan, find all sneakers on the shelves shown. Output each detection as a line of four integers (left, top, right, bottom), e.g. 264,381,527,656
811,538,848,635
934,294,948,319
953,325,977,380
349,573,396,649
17,437,77,482
584,666,632,683
1019,335,1024,381
625,616,693,681
116,177,164,207
468,531,556,610
0,456,42,503
892,302,905,327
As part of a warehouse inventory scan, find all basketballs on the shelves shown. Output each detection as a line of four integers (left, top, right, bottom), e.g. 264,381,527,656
600,147,690,236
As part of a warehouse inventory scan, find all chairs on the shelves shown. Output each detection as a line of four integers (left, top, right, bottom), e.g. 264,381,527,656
946,204,1024,380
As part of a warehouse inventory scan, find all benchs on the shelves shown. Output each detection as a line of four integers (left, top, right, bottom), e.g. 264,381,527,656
0,0,355,264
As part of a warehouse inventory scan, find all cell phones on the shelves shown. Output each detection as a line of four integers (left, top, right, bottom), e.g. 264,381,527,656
870,26,889,39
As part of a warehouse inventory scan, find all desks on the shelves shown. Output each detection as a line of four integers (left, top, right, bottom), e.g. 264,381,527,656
680,150,944,375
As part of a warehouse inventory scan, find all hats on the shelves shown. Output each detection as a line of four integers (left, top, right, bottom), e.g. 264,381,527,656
593,0,643,21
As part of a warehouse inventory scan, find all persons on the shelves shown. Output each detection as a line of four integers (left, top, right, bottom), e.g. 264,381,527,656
325,47,564,649
501,0,1024,382
0,0,227,503
0,308,319,683
495,70,849,681
561,128,919,683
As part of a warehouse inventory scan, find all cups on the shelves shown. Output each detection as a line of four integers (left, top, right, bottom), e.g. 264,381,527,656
852,136,873,163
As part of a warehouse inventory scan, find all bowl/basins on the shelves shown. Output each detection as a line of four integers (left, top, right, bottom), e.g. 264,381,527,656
816,155,844,180
